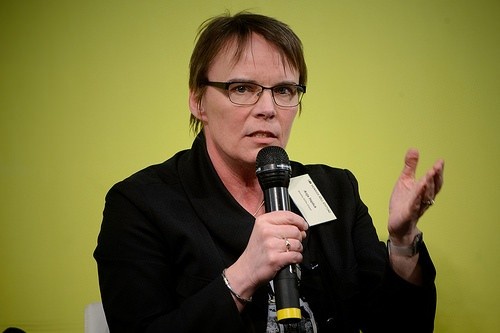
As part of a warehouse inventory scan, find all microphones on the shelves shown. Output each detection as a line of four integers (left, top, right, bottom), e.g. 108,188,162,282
256,146,302,324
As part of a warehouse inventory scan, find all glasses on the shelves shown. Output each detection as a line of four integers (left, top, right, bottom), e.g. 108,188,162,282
203,81,306,108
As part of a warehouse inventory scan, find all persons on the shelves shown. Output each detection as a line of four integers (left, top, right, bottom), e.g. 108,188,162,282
93,10,445,333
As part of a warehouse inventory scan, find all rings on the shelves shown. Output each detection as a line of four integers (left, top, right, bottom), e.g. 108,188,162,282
285,238,291,253
421,197,435,207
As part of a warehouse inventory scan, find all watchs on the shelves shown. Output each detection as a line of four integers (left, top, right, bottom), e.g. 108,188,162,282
387,230,424,256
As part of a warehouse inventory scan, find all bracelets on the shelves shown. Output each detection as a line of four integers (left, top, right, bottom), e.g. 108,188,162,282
223,268,252,303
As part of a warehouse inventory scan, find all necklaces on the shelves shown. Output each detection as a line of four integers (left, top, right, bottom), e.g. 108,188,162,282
253,202,265,216
